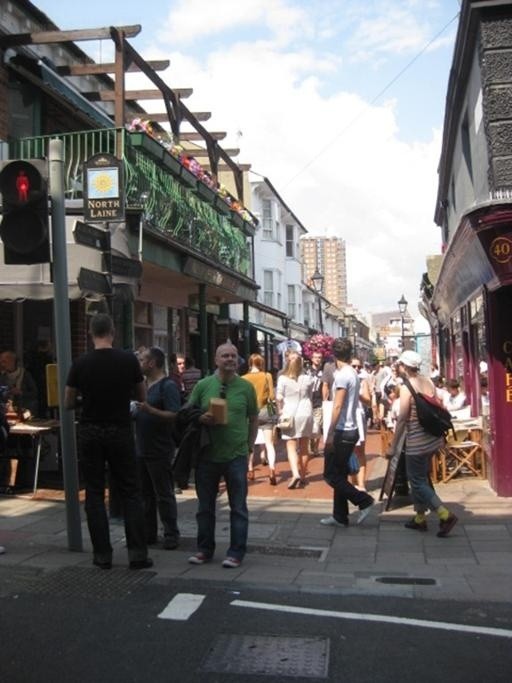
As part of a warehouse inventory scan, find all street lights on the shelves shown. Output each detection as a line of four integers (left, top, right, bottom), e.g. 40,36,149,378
310,263,328,337
396,291,408,354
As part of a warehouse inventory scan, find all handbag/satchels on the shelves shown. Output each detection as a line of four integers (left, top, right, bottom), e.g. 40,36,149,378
278,418,294,430
258,398,278,426
415,392,457,443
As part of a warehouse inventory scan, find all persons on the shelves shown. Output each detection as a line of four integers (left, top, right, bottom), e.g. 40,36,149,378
241,337,465,537
480,378,489,406
64,313,259,568
1,352,36,493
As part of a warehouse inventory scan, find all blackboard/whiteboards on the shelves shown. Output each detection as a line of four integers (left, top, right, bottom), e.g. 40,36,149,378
382,429,405,498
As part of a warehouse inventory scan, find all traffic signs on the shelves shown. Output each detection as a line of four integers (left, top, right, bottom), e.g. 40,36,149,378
101,251,143,280
72,219,114,253
75,265,114,292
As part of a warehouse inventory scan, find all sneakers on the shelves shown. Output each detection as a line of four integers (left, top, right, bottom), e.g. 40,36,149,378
405,516,427,531
321,517,348,528
269,470,276,486
247,472,254,481
92,560,112,568
130,558,154,569
222,557,241,568
357,500,375,524
164,540,179,551
437,512,458,537
189,552,213,564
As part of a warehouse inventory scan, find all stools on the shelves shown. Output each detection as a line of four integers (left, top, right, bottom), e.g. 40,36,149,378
444,441,481,483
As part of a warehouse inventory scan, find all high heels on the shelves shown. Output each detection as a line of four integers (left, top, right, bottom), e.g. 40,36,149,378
288,476,301,489
300,480,305,488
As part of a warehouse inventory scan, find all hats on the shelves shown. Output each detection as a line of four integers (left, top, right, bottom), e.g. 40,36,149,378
396,350,421,369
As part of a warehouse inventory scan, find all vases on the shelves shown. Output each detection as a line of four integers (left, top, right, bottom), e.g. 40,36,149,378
244,221,255,236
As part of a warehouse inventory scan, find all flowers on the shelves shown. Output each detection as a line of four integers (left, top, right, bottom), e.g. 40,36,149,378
128,117,257,228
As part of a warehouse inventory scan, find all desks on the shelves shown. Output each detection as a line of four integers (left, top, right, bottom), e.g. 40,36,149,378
7,420,79,496
431,421,488,483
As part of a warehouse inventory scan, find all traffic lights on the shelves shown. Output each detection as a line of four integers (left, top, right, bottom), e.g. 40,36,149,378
1,159,51,265
